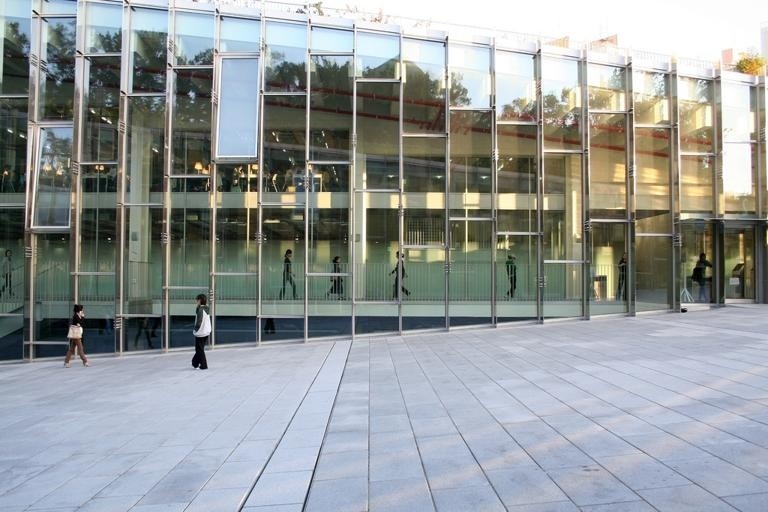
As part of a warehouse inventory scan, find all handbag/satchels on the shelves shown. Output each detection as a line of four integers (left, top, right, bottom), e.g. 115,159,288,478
67,325,83,339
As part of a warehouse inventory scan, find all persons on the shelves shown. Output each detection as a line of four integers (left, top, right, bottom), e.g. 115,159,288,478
134,317,154,349
279,250,298,300
616,253,626,300
389,251,411,301
325,256,346,301
696,253,712,300
64,305,91,368
191,294,211,369
506,254,517,298
0,249,16,297
264,319,275,335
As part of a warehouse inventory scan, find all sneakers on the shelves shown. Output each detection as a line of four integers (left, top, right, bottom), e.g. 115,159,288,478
64,363,70,368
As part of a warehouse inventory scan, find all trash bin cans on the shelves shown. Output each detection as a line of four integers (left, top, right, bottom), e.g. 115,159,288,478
592,275,606,300
